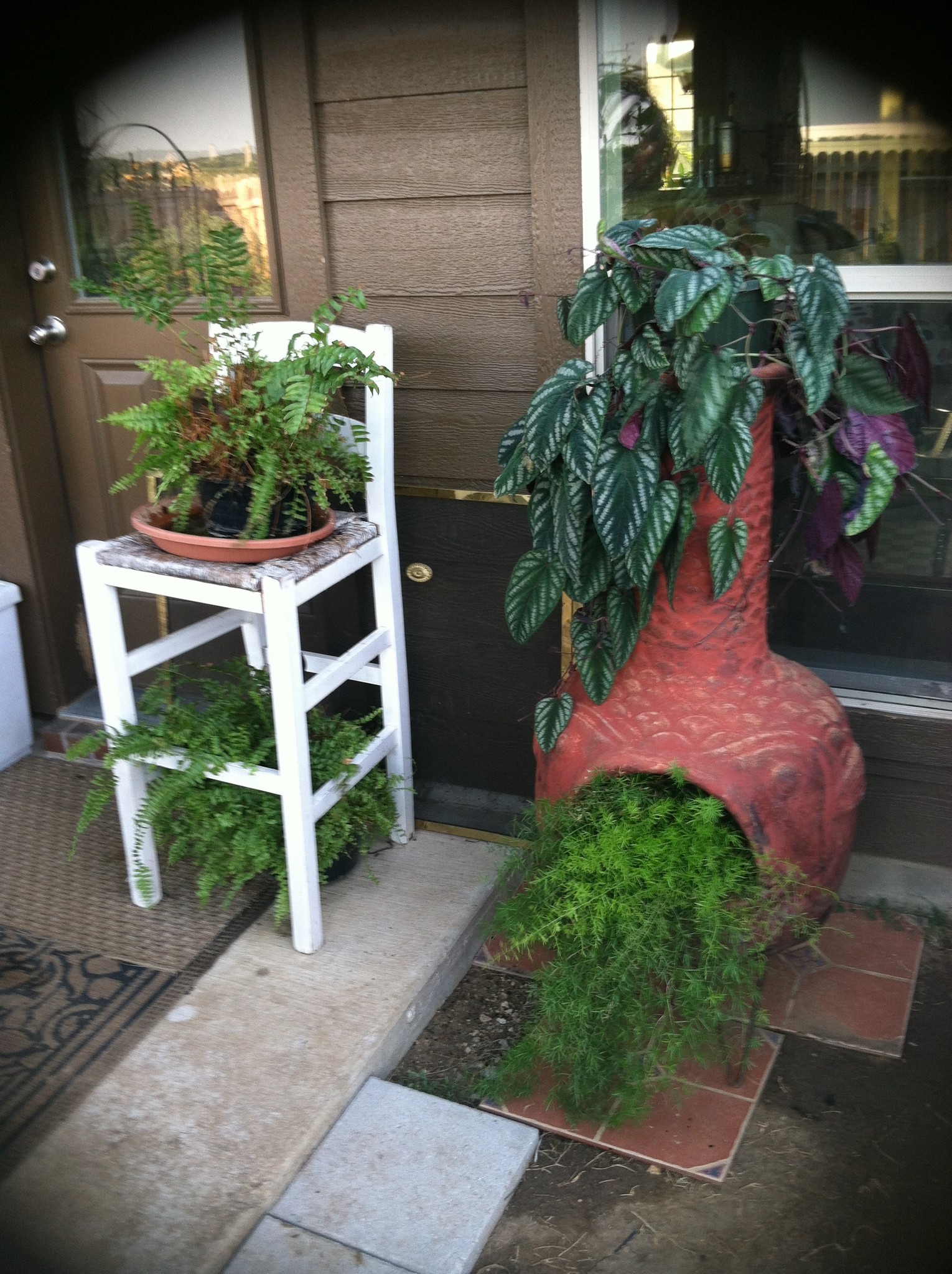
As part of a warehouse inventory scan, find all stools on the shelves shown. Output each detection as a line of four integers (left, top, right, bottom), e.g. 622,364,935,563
74,313,415,954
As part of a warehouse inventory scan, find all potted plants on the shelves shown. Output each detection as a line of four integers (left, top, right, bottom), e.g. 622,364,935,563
62,658,406,932
397,215,952,1127
65,147,403,566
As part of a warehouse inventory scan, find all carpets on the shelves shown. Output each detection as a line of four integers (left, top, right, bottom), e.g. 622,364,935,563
0,751,278,1180
